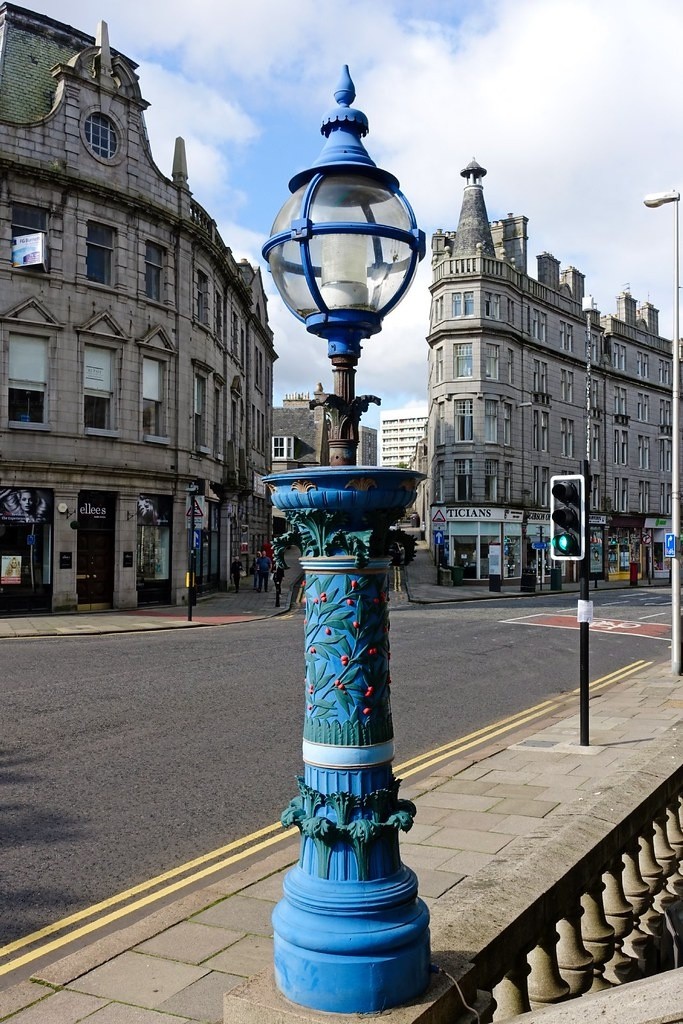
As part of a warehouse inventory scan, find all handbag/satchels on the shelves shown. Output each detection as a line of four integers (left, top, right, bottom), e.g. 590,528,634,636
249,558,255,575
239,567,247,578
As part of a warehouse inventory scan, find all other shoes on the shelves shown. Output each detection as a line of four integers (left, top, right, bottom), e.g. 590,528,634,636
265,590,269,592
235,591,239,593
256,589,261,593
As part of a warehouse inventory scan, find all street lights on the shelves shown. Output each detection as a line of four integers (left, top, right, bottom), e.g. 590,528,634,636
257,65,434,1015
641,186,683,675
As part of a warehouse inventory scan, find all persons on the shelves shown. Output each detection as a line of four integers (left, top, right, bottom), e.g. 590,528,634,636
5,557,21,576
232,557,243,592
137,494,158,526
252,551,272,593
17,486,47,524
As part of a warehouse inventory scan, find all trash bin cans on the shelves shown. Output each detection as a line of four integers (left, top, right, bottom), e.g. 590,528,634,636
521,567,537,592
412,514,420,528
448,566,464,586
550,568,562,591
489,574,500,592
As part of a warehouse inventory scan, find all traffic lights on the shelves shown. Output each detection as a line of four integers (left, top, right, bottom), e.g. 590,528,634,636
624,538,628,545
546,475,586,561
612,538,616,545
